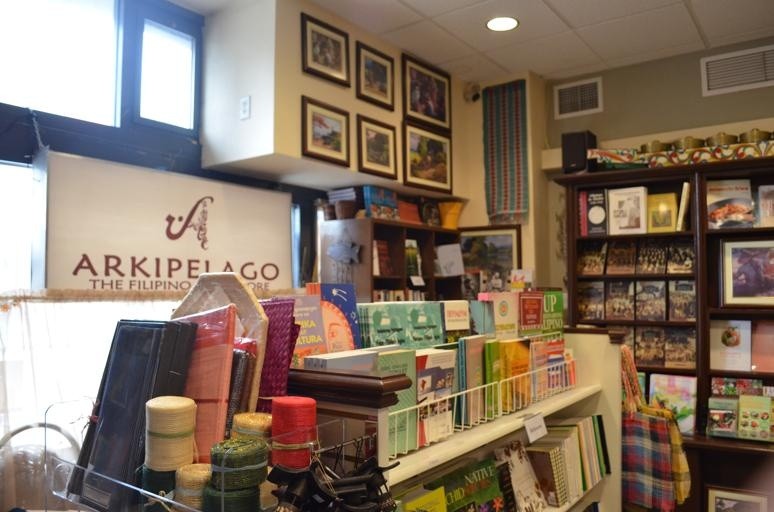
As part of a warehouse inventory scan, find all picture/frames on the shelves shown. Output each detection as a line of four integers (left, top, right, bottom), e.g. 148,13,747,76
357,114,397,180
705,483,772,512
356,40,394,111
300,12,349,87
401,52,451,139
458,224,522,269
302,95,349,167
719,237,774,309
403,120,452,194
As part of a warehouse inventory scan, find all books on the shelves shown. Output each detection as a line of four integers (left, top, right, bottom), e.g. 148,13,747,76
172,279,574,461
577,178,773,238
398,415,613,511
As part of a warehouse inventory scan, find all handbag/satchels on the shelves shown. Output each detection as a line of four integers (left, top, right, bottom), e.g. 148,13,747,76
618,343,694,512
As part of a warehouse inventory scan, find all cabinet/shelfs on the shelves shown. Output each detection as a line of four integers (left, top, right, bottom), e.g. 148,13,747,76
553,140,774,511
46,344,622,512
315,207,461,302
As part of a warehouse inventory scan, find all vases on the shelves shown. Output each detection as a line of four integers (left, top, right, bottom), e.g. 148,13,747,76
323,205,335,220
335,200,357,219
